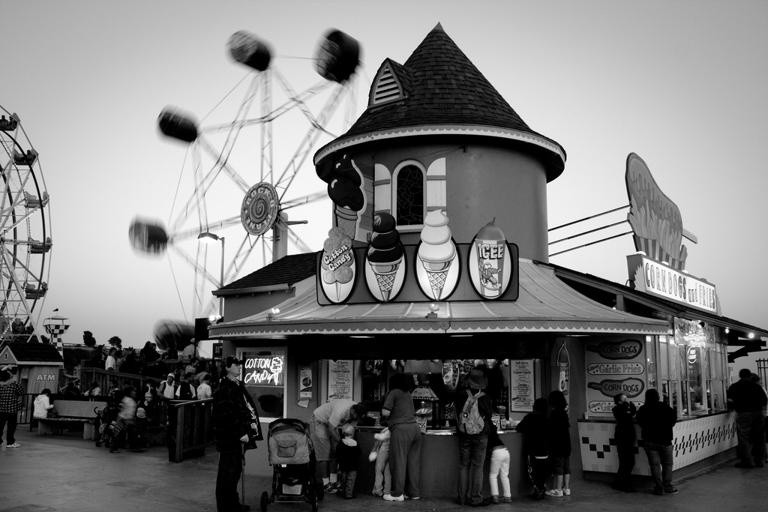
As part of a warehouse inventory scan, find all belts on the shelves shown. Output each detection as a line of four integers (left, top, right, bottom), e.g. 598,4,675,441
311,413,328,426
390,422,418,426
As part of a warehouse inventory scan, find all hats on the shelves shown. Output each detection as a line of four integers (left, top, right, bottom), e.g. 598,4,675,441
221,355,244,366
468,370,488,390
168,373,174,377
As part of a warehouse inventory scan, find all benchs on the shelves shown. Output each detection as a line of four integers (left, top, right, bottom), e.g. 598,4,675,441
33,398,108,439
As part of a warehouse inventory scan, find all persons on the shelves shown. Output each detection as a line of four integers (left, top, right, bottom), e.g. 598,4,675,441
726,368,768,469
33,342,212,453
635,388,679,496
611,392,638,493
0,363,25,448
213,355,264,512
310,375,573,506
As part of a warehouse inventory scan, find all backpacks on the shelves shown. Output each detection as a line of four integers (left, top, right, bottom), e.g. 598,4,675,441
458,389,485,434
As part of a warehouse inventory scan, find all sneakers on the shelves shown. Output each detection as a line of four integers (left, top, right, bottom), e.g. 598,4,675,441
405,496,420,499
546,489,571,497
7,441,21,447
653,488,680,495
383,494,404,502
323,482,342,494
493,497,513,504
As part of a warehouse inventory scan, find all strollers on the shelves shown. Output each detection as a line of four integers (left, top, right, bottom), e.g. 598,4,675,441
259,418,324,512
